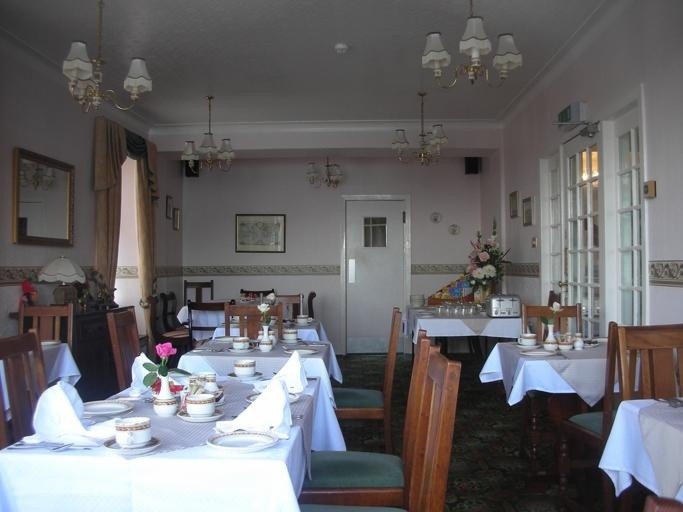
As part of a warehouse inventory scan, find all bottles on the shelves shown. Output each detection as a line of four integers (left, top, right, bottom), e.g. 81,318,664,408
256,331,263,343
573,332,584,350
556,335,573,342
268,331,277,345
238,293,263,305
179,372,225,406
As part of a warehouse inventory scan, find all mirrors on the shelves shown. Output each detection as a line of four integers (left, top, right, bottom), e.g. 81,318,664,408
10,145,74,249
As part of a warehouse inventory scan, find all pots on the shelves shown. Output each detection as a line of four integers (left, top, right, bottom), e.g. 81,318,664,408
486,294,522,318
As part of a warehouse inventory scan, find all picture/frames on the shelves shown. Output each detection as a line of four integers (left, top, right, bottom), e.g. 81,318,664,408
521,195,534,227
165,195,180,230
233,212,286,254
509,190,518,218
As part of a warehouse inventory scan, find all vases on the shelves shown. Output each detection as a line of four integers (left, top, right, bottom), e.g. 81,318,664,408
540,315,558,352
154,367,179,419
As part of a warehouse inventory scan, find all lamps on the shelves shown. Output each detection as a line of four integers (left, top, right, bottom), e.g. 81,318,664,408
417,0,526,93
17,159,57,193
58,1,154,116
180,93,235,178
35,254,87,313
302,154,344,192
386,90,449,167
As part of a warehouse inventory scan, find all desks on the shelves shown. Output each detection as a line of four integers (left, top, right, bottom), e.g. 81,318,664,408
176,341,347,454
480,342,627,500
404,299,527,381
0,375,321,512
600,399,683,512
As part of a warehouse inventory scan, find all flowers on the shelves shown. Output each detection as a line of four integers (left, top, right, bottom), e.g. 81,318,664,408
550,302,562,312
457,228,513,291
152,340,175,370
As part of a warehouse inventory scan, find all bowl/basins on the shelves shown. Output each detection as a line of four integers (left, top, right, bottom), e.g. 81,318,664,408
408,294,424,310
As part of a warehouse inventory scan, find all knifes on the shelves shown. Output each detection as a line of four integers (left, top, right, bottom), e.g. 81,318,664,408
67,447,92,451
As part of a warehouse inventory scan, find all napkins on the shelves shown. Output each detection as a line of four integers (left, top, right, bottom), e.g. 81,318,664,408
216,376,292,440
127,351,164,389
263,350,309,393
27,380,102,446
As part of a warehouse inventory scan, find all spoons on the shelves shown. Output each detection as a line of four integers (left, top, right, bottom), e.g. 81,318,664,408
4,442,74,453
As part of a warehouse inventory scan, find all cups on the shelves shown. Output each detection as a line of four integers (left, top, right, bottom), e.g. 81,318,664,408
296,314,312,326
557,341,573,351
517,333,538,347
281,329,296,340
204,336,256,353
113,416,152,449
544,341,557,351
231,359,256,378
436,302,480,317
184,393,216,419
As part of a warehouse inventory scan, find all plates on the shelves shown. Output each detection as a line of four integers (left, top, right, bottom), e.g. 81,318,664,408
102,436,162,455
281,348,320,356
39,339,62,346
175,406,226,423
226,372,262,381
219,315,276,328
520,351,559,357
203,429,279,455
242,390,300,405
516,345,539,351
81,398,133,416
584,341,599,348
280,339,299,344
414,310,431,315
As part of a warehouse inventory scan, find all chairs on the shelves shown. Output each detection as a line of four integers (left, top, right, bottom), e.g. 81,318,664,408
146,278,343,381
557,323,660,508
330,306,403,454
2,328,53,455
618,320,681,508
297,329,462,511
17,300,74,369
519,302,597,457
105,305,147,390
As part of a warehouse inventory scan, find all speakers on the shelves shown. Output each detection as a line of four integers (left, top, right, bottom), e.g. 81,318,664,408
464,158,479,174
185,161,199,176
